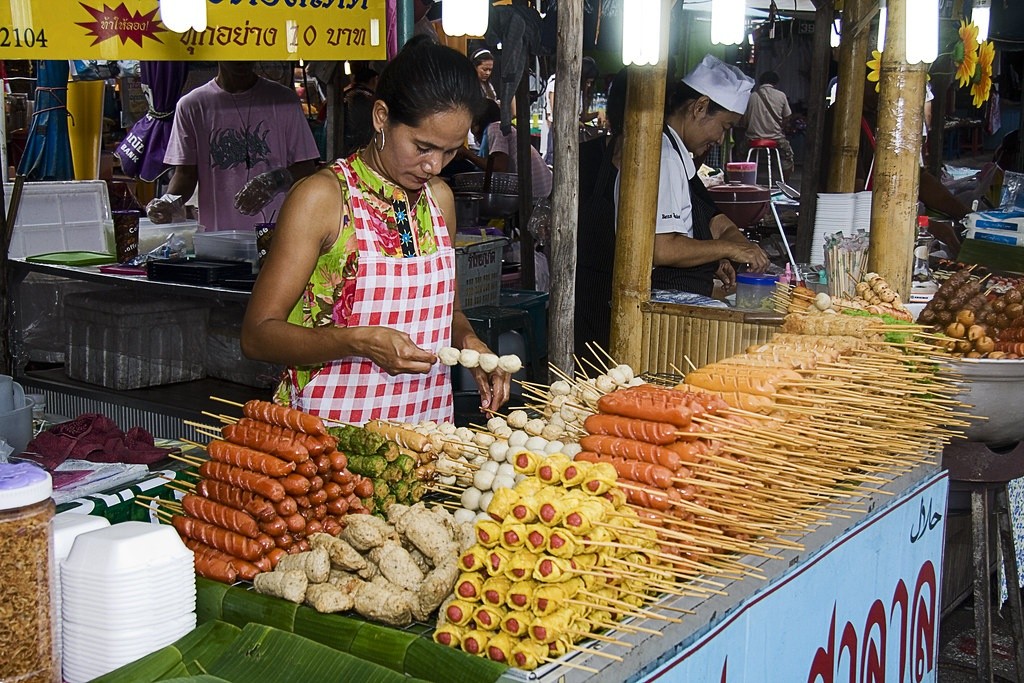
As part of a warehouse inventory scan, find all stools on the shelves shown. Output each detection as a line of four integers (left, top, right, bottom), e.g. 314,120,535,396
746,136,786,189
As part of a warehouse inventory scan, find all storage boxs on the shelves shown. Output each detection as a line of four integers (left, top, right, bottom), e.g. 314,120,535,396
10,268,282,394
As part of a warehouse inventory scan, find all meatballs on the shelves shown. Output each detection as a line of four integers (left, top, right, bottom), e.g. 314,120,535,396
386,344,652,523
814,273,905,319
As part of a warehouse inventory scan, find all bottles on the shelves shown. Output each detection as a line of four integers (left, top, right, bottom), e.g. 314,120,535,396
0,462,62,683
735,273,776,312
912,216,935,281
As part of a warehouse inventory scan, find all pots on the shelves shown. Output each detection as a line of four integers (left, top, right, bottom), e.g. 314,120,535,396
706,183,772,229
927,357,1024,448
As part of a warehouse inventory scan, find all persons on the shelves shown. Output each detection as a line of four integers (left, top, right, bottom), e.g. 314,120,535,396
238,32,515,437
460,96,553,201
544,57,601,170
464,47,517,151
345,69,380,145
146,61,322,234
818,65,975,258
611,52,771,297
732,70,796,184
918,74,936,171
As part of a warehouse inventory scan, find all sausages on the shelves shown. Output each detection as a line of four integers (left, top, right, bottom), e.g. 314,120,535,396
785,283,816,314
993,325,1024,357
169,398,374,585
359,413,440,491
452,344,925,671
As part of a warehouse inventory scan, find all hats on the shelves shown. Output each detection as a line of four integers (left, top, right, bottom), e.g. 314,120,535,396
681,52,756,116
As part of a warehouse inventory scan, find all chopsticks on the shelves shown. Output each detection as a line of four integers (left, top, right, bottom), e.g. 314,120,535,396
822,242,868,300
